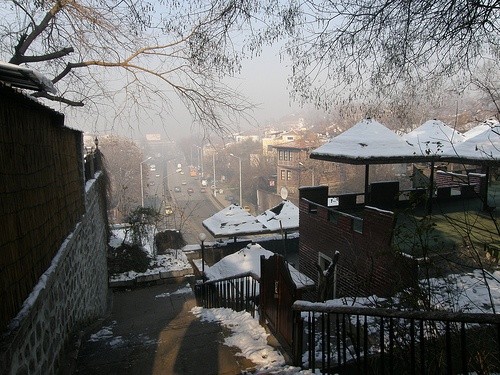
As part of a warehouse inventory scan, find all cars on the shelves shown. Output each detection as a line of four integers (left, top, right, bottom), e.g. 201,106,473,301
165,168,240,215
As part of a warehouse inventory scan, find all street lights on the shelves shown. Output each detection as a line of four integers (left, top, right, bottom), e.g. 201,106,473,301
230,154,242,207
140,157,152,208
198,232,207,283
